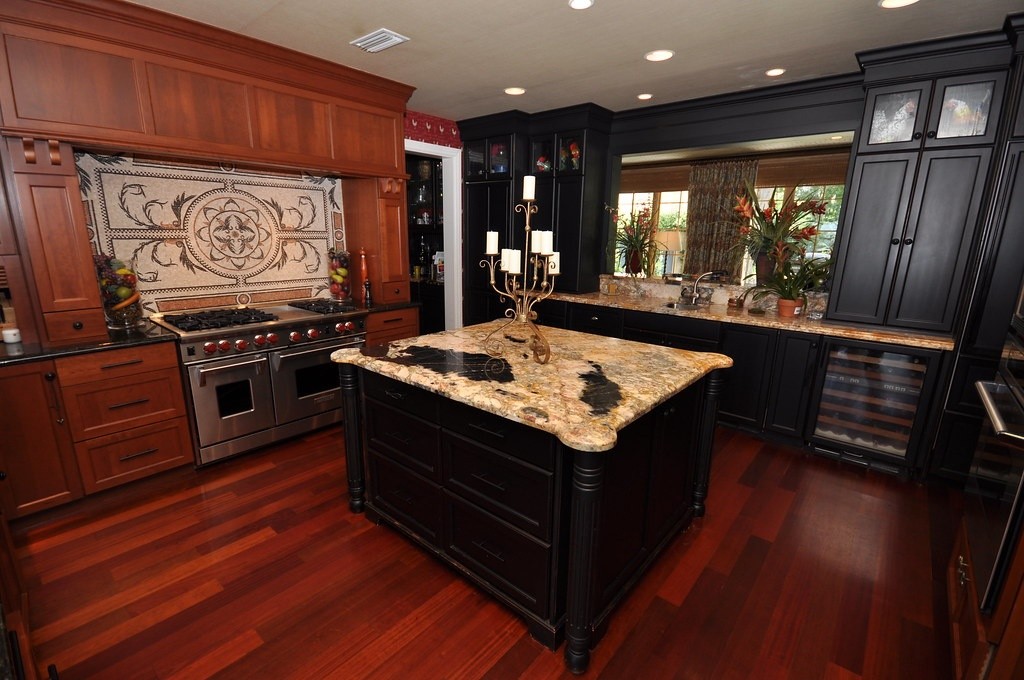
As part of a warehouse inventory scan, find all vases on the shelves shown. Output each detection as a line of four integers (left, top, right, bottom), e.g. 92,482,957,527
756,256,773,287
625,249,643,274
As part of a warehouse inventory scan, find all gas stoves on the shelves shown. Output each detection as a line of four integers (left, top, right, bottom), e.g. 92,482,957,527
149,295,366,340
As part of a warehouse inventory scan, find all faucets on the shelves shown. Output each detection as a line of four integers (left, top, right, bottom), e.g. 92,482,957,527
681,270,728,305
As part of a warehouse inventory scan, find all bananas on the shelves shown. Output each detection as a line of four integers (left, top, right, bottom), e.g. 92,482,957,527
101,269,134,285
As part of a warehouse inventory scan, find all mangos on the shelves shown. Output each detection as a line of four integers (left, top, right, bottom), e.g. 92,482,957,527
328,267,348,284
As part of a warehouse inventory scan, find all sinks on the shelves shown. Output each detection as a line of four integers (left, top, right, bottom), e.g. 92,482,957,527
657,300,712,311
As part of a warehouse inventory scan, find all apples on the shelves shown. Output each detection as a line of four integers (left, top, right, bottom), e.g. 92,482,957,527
124,274,136,284
116,287,132,299
330,260,340,270
330,284,347,300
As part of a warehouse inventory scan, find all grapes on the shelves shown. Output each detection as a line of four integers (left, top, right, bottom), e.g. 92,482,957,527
91,251,143,322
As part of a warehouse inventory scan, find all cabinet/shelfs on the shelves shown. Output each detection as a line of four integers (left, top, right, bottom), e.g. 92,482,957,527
857,64,1013,155
404,154,444,269
339,363,723,675
532,300,822,448
0,21,419,522
821,144,994,338
463,127,607,295
943,517,995,680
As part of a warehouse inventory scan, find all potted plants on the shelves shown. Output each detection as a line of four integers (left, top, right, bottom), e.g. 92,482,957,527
735,254,832,317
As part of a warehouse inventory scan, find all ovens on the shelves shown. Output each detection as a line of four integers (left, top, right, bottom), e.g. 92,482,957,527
181,338,364,463
962,275,1024,613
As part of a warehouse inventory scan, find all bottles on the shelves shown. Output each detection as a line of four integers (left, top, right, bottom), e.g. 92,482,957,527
415,185,432,204
422,212,429,224
418,160,432,179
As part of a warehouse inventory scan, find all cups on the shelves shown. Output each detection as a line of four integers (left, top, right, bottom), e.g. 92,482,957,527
417,218,424,224
413,266,420,280
6,343,24,356
2,328,21,343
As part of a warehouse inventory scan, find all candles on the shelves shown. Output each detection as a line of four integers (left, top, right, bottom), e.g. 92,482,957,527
523,176,535,199
531,229,543,253
501,249,512,270
548,252,559,274
540,230,553,254
509,249,520,273
487,231,498,254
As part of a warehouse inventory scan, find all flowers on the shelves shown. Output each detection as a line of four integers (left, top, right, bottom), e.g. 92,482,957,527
603,192,670,282
707,176,828,263
